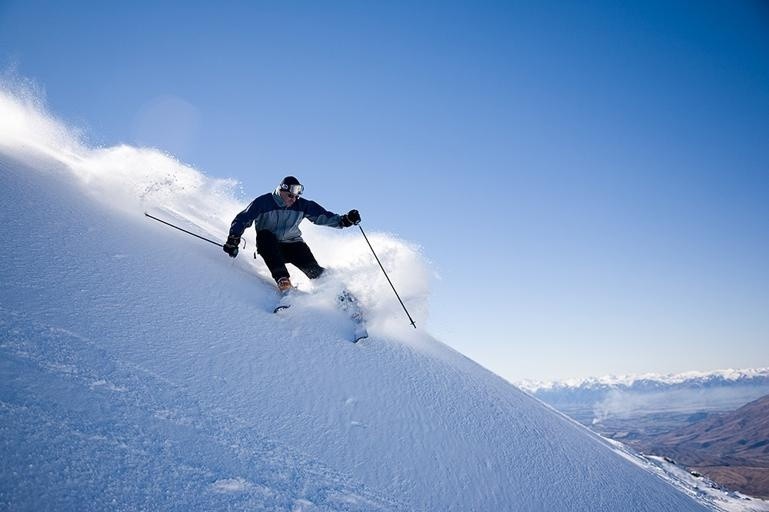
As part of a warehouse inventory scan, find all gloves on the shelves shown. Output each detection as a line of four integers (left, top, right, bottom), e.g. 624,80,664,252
223,234,239,258
340,209,360,227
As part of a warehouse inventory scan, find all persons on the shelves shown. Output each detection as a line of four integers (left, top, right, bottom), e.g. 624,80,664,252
223,175,366,327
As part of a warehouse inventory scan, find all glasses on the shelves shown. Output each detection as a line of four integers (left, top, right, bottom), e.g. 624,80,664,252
287,193,298,199
281,184,305,196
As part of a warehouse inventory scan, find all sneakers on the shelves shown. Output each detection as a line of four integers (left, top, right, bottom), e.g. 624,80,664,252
278,275,298,302
350,311,361,323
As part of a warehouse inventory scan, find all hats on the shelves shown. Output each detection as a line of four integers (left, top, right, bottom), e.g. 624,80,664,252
280,177,300,186
